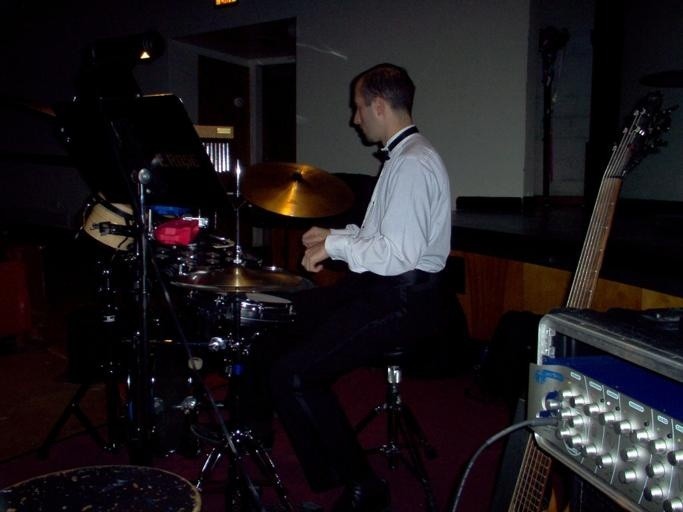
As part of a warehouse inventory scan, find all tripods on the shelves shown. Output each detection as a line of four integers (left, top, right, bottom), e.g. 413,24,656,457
193,324,296,512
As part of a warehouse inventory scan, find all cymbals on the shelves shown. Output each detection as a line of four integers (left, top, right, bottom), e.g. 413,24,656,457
239,162,351,219
170,269,301,292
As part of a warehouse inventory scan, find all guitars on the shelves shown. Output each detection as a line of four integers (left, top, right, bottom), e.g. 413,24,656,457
509,89,680,509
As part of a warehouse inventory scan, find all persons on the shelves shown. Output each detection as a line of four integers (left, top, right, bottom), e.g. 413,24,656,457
223,63,452,512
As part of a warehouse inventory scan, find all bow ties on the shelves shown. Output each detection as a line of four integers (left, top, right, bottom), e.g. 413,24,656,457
372,150,390,162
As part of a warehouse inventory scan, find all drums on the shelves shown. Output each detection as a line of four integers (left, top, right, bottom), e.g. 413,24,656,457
155,235,239,283
205,294,296,337
82,192,136,253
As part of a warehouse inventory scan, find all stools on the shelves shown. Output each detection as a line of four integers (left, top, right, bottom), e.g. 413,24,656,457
340,296,469,512
